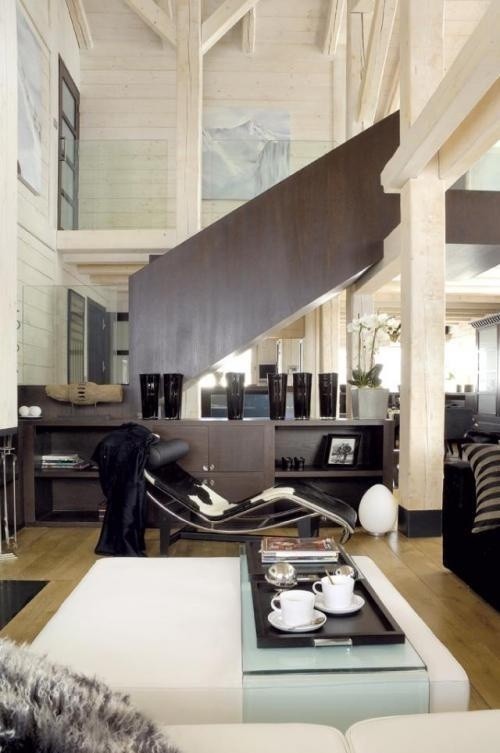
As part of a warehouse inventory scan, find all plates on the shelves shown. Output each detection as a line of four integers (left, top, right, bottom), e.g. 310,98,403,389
315,594,367,616
242,537,408,650
265,608,330,633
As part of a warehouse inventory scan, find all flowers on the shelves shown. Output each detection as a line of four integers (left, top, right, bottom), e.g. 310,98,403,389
346,308,401,388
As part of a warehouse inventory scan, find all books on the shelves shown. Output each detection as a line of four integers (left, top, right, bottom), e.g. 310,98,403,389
260,535,340,566
40,453,91,470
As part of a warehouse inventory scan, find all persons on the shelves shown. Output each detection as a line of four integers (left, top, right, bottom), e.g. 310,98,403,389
342,444,352,462
335,443,344,464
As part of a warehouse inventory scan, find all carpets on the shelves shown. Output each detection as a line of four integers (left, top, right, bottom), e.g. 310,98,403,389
0,580,52,634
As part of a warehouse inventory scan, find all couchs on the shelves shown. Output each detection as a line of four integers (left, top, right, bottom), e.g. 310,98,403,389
155,709,500,753
442,442,500,612
25,556,471,725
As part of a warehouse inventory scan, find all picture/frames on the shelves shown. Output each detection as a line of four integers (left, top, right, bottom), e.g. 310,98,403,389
392,394,400,408
327,433,361,470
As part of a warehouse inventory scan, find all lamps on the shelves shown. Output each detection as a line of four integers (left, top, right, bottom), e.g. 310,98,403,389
358,484,396,535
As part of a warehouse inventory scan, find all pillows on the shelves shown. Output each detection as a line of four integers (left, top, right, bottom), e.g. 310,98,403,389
461,442,500,533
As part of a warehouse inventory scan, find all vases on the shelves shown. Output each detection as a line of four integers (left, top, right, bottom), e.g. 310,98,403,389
347,390,390,418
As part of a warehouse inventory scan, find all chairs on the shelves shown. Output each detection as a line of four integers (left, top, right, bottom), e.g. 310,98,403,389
144,462,360,555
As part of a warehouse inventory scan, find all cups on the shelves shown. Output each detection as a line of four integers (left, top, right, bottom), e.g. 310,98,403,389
311,574,355,611
270,589,318,628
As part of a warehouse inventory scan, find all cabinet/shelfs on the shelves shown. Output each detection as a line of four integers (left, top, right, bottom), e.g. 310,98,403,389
470,312,500,432
445,393,477,439
22,421,395,527
